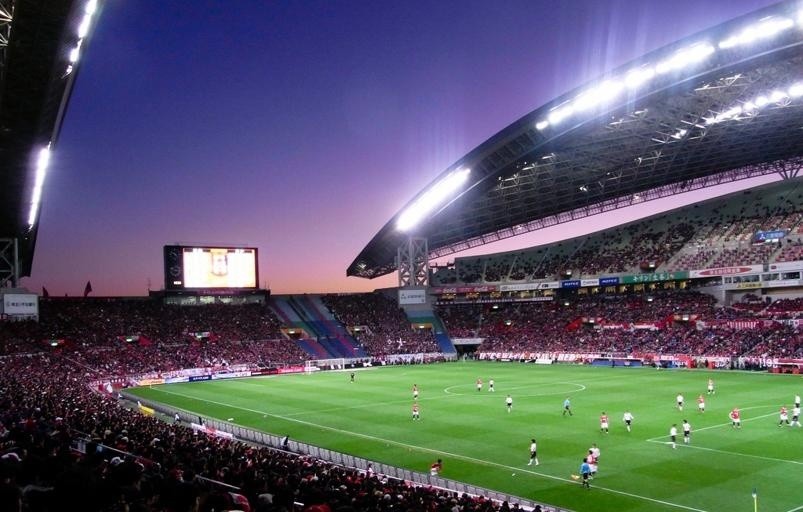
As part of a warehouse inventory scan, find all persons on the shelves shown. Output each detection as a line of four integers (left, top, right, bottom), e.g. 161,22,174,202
600,412,609,434
580,443,600,490
728,406,741,428
431,194,802,370
622,409,634,432
562,396,573,416
476,377,483,392
0,383,543,512
669,378,715,449
0,290,431,387
488,378,495,392
779,393,802,427
